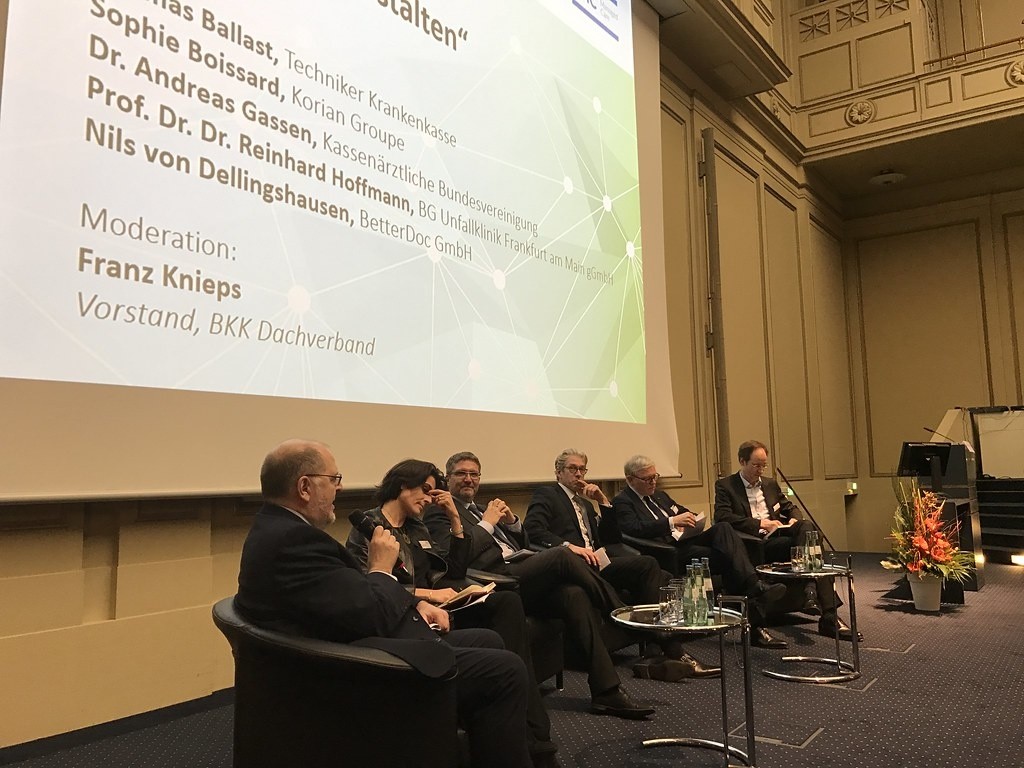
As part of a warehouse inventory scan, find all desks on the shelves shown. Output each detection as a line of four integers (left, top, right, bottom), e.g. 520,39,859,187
609,596,757,768
755,561,862,684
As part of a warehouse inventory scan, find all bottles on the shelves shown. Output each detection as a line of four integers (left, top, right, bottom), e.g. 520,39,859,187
683,557,716,626
805,529,824,573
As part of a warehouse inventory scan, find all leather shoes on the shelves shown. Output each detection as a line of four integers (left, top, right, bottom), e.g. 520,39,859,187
818,616,863,641
678,652,721,678
753,583,787,601
741,627,788,649
633,654,694,681
591,683,655,716
799,586,822,615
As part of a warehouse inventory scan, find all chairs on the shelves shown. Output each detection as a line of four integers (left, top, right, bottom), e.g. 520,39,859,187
464,534,676,692
213,597,469,768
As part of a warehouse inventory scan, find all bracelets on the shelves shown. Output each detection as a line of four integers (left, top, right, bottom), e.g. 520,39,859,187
450,525,463,535
428,590,433,599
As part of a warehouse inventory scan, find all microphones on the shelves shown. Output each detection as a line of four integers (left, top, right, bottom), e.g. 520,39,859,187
924,426,955,442
348,509,411,577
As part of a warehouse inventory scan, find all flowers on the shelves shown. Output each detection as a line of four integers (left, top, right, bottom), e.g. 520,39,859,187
881,477,977,584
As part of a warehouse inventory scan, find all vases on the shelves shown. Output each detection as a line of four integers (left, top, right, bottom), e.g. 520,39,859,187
907,573,944,610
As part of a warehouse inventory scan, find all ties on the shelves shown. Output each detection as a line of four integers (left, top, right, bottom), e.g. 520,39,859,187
573,494,595,552
643,495,666,519
469,504,518,553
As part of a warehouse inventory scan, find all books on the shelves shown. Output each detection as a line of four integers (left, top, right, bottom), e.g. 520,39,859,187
438,581,497,612
764,524,795,542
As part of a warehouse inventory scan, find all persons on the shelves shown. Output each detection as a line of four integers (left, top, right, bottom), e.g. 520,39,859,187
236,440,559,768
610,455,789,649
714,440,864,641
345,458,534,669
423,451,656,718
522,448,722,681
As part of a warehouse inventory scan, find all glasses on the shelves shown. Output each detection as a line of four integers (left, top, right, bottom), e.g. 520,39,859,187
448,471,480,480
745,461,770,471
632,472,660,483
420,482,437,499
298,472,342,486
561,465,588,475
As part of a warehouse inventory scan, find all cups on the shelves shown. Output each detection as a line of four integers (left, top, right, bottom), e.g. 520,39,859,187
658,577,687,626
790,545,806,573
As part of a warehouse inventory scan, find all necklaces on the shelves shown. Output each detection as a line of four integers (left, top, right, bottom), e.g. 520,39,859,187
383,507,411,544
